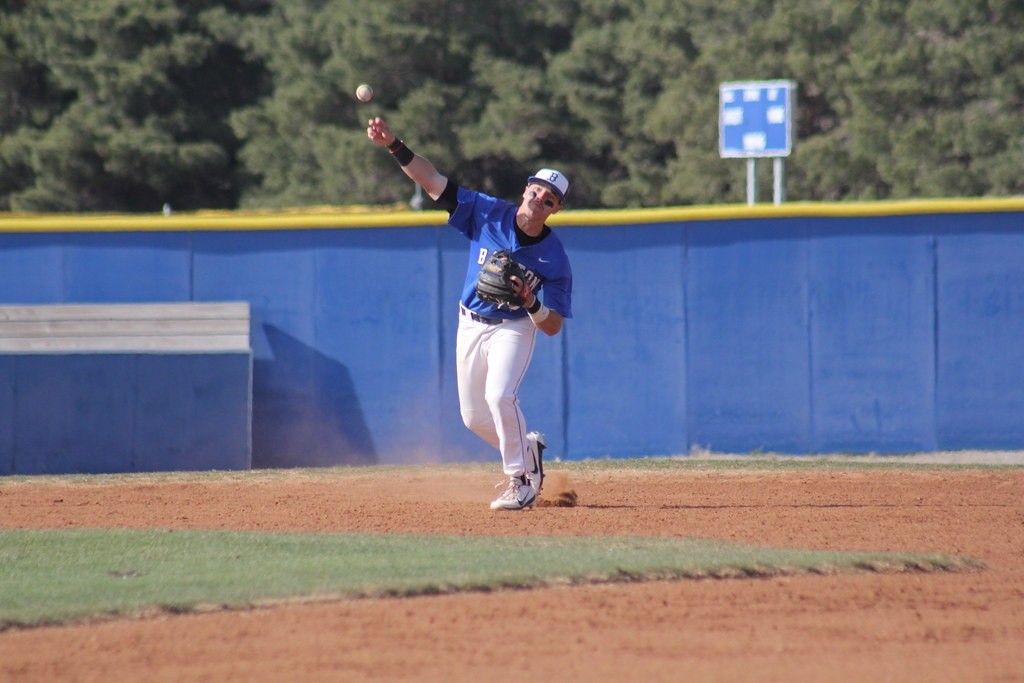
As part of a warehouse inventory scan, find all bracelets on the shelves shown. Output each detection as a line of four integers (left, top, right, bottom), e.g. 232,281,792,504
389,141,403,154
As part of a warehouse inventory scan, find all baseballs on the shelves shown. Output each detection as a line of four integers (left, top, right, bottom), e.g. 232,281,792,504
356,84,374,103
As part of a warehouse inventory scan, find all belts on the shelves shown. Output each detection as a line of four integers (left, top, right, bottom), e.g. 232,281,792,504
460,307,503,326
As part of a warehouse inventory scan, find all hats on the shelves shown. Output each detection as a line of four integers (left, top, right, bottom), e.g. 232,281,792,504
528,168,571,204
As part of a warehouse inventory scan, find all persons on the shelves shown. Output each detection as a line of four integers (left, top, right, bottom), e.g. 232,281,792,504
366,117,572,510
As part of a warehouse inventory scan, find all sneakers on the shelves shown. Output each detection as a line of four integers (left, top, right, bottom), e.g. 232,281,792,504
489,475,537,511
526,431,547,496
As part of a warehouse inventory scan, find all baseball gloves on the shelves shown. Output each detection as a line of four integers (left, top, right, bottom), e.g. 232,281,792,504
475,247,532,312
